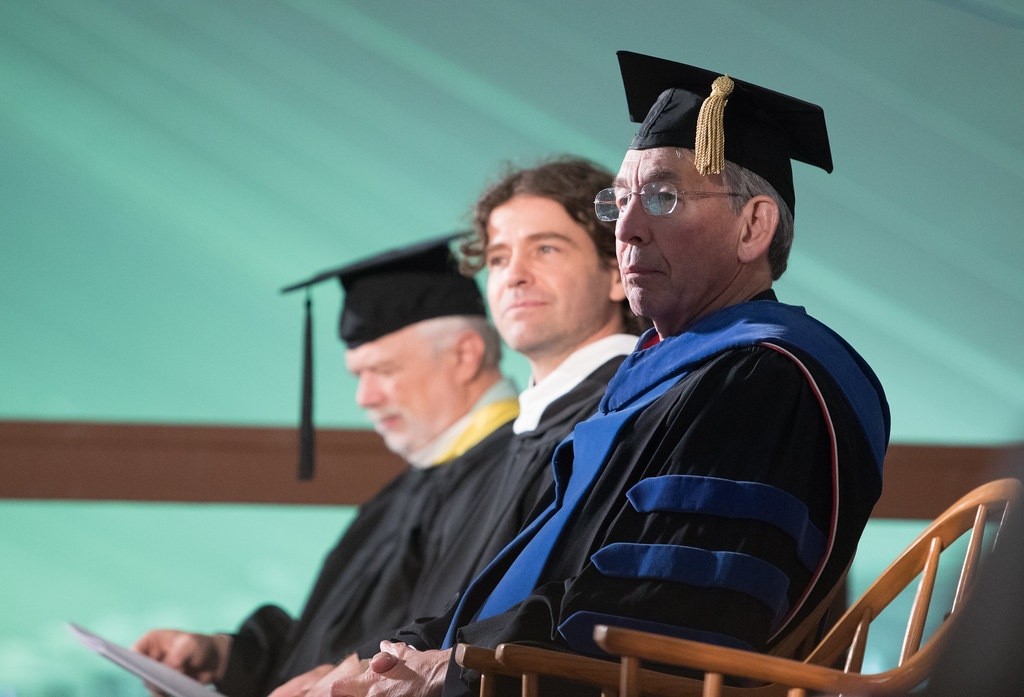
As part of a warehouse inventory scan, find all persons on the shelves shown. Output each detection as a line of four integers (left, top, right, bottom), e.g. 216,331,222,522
134,232,522,697
331,50,891,697
270,156,654,697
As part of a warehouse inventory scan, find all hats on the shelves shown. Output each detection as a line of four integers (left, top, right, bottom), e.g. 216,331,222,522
279,229,475,482
617,50,833,220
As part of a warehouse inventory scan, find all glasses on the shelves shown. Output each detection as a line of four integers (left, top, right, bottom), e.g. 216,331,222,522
593,181,752,222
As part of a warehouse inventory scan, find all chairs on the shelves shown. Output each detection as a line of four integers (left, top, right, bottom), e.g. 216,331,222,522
450,473,1024,697
